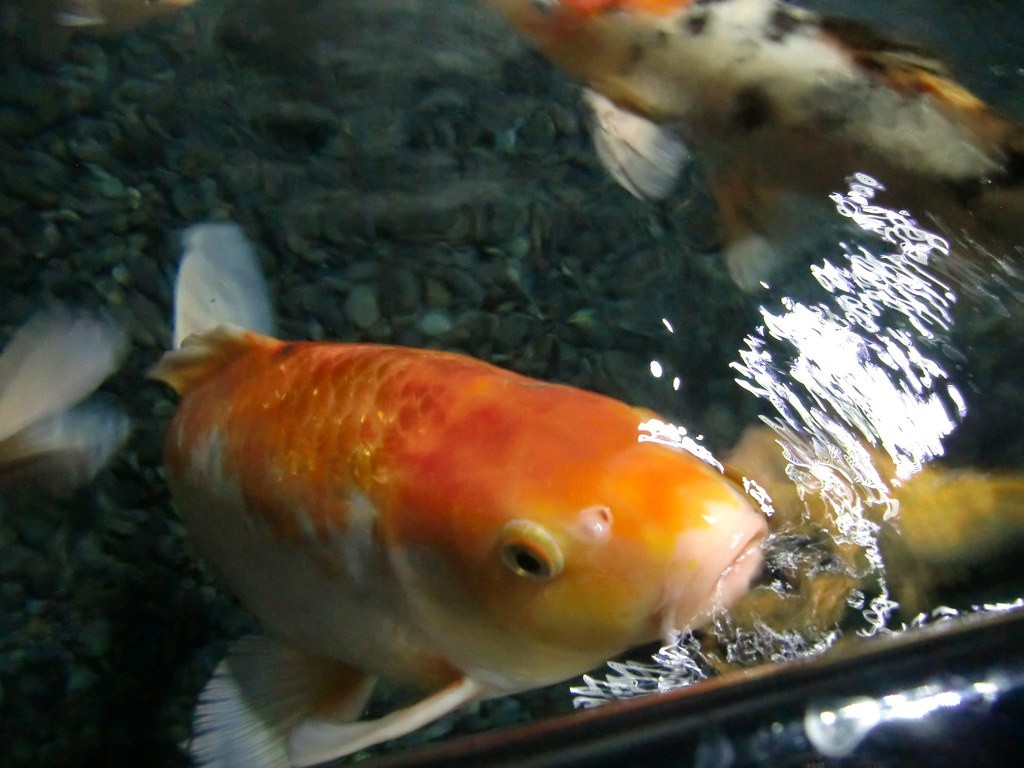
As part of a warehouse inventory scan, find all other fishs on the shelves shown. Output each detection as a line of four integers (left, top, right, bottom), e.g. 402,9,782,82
0,217,1023,768
475,0,1022,293
